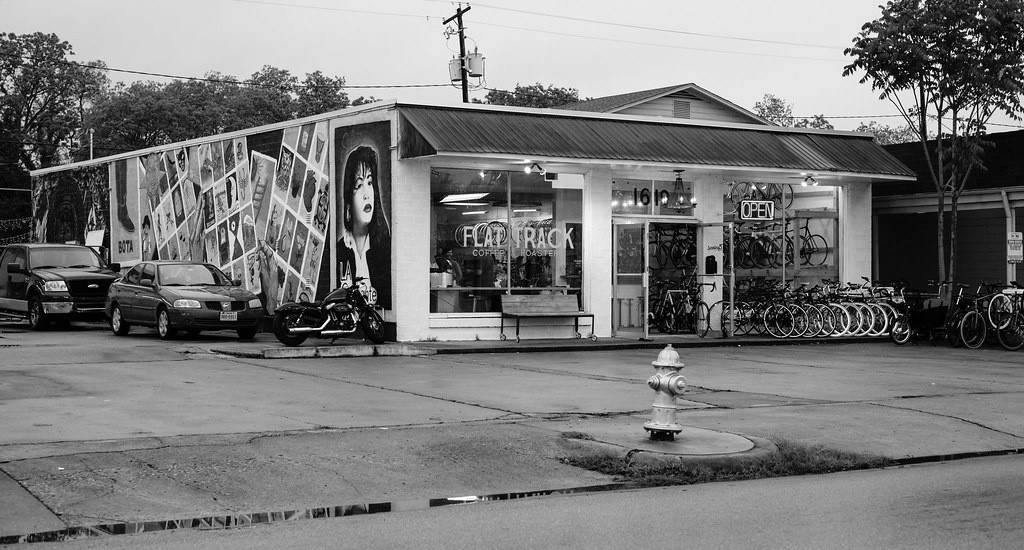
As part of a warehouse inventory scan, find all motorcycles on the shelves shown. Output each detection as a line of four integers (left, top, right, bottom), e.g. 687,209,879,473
272,277,388,347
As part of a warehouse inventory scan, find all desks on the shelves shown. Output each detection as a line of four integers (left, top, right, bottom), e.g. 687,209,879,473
433,286,581,312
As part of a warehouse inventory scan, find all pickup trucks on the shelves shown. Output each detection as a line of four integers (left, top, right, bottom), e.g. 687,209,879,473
0,241,125,332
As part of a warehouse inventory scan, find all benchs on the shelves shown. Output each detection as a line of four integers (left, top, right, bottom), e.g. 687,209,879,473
501,294,594,342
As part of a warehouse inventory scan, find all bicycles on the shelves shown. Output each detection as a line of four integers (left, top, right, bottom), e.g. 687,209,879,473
616,180,1024,352
434,220,583,313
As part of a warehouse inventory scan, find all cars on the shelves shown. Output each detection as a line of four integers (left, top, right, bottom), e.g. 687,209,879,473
104,259,266,341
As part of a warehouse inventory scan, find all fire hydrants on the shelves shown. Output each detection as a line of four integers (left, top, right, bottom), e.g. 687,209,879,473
643,343,690,442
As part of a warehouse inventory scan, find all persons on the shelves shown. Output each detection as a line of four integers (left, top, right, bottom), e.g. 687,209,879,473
115,159,135,232
336,146,391,310
442,248,454,259
